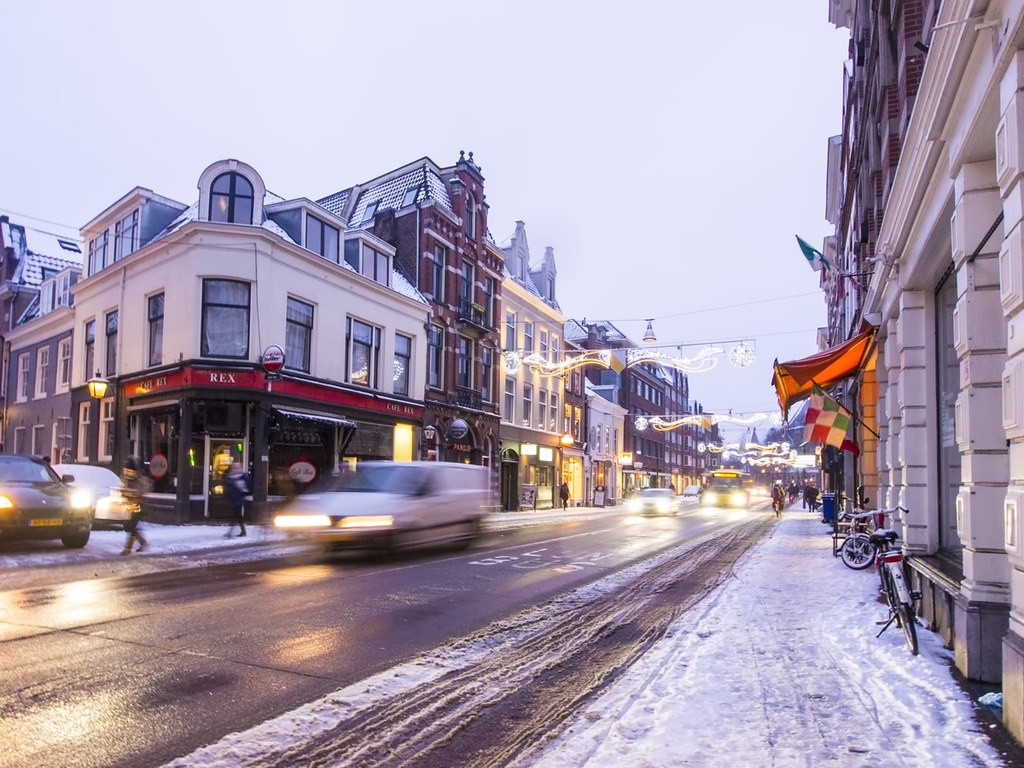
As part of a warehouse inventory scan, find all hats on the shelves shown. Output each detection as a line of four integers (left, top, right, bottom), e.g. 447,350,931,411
125,458,136,470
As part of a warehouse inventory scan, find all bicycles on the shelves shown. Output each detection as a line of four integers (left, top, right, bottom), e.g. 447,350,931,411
836,512,875,572
772,492,782,519
705,469,754,507
839,507,923,653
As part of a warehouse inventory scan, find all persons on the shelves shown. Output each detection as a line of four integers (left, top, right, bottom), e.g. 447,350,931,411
559,482,570,510
119,461,149,556
224,461,252,539
668,483,675,493
769,478,820,514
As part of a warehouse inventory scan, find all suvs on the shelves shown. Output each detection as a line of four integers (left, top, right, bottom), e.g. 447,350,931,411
290,460,488,550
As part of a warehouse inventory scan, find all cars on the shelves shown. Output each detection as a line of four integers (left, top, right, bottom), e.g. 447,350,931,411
684,484,702,499
631,487,677,517
2,455,90,552
47,461,137,532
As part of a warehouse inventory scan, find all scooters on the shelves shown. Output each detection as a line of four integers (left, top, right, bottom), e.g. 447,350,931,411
785,487,797,503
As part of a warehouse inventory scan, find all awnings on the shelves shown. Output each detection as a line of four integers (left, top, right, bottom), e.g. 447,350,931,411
771,325,877,425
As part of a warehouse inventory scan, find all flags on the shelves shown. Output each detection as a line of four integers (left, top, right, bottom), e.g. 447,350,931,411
796,235,830,271
802,382,860,458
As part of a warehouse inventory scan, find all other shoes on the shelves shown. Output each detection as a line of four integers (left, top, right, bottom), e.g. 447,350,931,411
223,533,247,538
119,546,151,555
